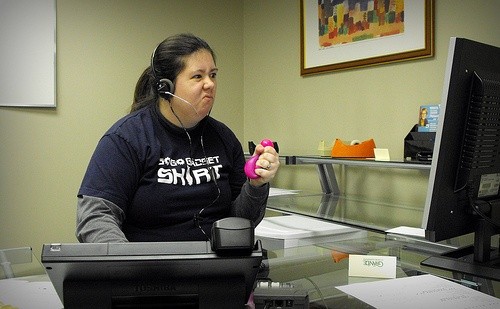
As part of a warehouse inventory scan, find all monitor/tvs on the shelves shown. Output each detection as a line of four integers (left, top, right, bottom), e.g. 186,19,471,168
42,241,263,309
420,36,500,282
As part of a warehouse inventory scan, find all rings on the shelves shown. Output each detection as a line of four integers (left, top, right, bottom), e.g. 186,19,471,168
266,161,271,170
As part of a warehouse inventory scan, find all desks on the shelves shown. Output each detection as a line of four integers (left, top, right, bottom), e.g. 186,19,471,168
0,232,500,309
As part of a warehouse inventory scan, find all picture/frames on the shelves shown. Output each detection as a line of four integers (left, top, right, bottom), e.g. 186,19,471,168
299,0,434,76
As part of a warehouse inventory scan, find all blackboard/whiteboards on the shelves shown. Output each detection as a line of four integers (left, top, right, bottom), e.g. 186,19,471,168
0,0,58,109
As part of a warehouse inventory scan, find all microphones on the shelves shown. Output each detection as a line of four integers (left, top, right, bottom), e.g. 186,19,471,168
165,91,198,115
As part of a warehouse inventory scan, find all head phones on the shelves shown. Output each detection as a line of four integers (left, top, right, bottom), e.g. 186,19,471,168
151,45,174,99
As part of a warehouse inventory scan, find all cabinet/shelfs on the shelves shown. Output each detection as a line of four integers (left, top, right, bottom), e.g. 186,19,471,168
404,124,436,162
243,151,440,237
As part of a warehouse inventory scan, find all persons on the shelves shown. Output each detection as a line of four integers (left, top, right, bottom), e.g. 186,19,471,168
75,34,280,309
